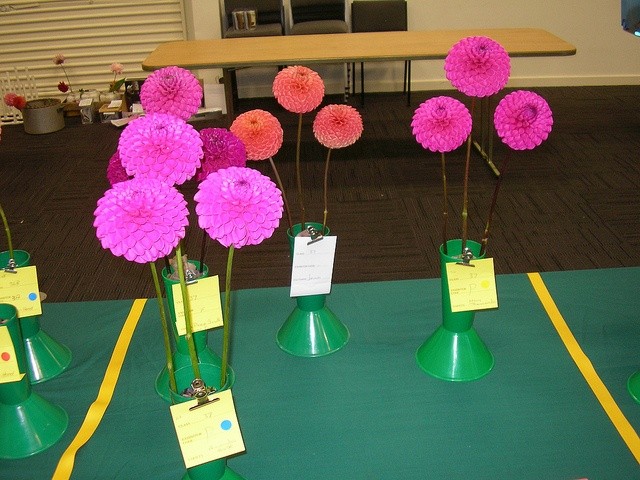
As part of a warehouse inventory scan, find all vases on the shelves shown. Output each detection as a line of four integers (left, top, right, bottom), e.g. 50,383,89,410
109,62,125,94
229,64,363,234
412,39,553,257
106,67,247,276
58,81,91,94
4,93,51,110
53,53,78,102
94,114,284,394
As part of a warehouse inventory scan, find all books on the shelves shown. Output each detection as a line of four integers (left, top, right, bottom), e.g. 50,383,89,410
63,94,128,117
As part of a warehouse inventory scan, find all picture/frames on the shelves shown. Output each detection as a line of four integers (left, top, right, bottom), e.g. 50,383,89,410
142,29,576,180
0,265,640,480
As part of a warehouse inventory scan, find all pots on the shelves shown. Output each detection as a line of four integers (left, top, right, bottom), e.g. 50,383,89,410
15,99,71,137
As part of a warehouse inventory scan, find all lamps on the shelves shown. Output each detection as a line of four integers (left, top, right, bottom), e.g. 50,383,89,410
290,0,351,105
351,1,414,107
222,0,287,109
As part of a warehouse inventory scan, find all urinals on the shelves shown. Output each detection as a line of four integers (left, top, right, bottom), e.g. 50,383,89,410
0,326,25,385
0,266,42,320
173,276,222,336
445,258,499,313
169,390,245,469
290,236,337,298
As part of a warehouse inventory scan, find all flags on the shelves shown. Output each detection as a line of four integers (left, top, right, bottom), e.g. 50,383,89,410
275,223,351,358
1,303,69,459
100,91,121,102
155,260,235,404
67,91,82,102
167,364,244,479
0,249,72,385
82,89,100,102
416,238,495,382
